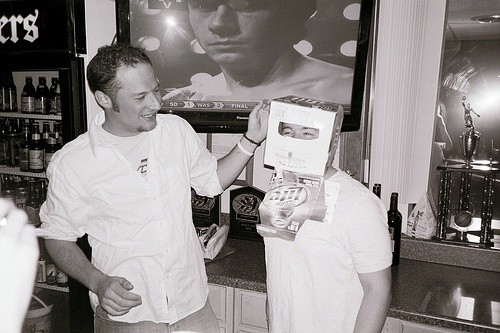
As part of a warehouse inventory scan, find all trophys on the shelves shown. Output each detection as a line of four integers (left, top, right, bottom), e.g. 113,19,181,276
460,96,481,168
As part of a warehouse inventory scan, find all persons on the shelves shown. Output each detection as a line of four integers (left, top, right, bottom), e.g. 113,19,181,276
263,123,392,333
162,0,354,104
39,45,271,333
0,198,39,333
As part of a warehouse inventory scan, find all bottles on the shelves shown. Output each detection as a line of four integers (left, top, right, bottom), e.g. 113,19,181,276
387,192,402,267
373,184,381,199
20,76,36,114
35,238,70,288
0,174,49,228
48,76,58,116
3,72,18,113
0,117,64,173
56,85,62,115
34,76,49,115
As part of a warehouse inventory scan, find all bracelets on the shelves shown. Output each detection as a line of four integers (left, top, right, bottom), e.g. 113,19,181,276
238,132,261,156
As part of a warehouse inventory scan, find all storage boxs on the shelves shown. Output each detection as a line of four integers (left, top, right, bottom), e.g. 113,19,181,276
252,94,343,239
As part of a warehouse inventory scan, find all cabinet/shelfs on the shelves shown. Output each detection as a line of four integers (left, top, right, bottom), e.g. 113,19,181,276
208,282,469,333
0,55,94,333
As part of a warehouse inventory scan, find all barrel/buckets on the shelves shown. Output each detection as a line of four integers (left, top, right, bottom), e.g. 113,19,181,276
22,292,56,333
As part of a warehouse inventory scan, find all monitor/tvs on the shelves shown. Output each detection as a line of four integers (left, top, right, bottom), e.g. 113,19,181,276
113,0,375,135
